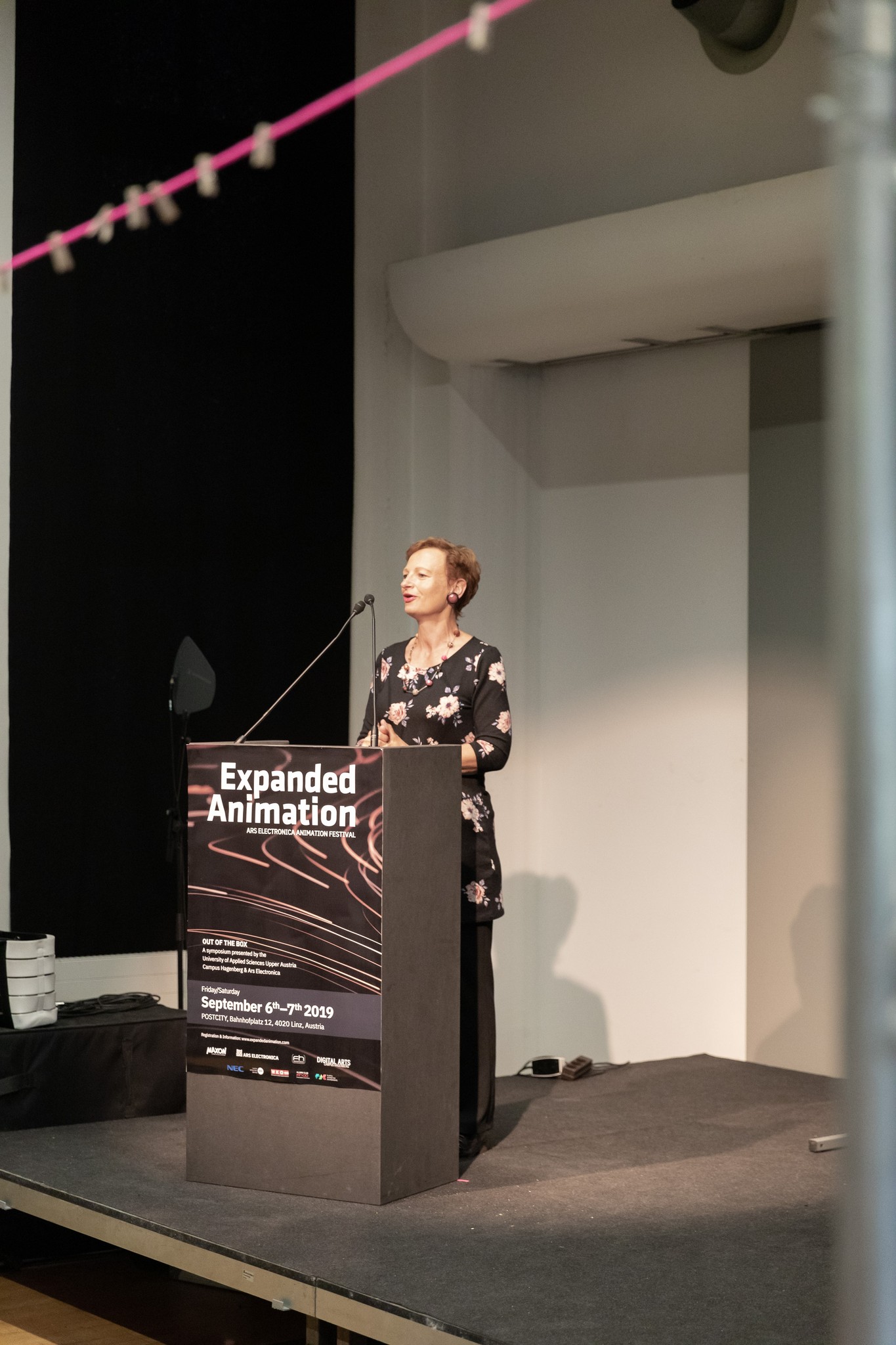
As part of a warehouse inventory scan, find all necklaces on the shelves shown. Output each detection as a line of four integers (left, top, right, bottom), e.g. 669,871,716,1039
402,630,460,695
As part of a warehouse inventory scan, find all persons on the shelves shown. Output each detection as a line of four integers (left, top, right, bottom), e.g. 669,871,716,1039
355,536,512,1160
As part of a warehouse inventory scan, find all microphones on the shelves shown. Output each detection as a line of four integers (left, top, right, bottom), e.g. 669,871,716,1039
233,601,366,743
364,594,378,747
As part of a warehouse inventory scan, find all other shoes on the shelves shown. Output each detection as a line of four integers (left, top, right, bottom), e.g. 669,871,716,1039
459,1128,490,1156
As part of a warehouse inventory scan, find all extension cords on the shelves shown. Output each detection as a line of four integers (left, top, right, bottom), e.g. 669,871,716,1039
562,1055,592,1080
